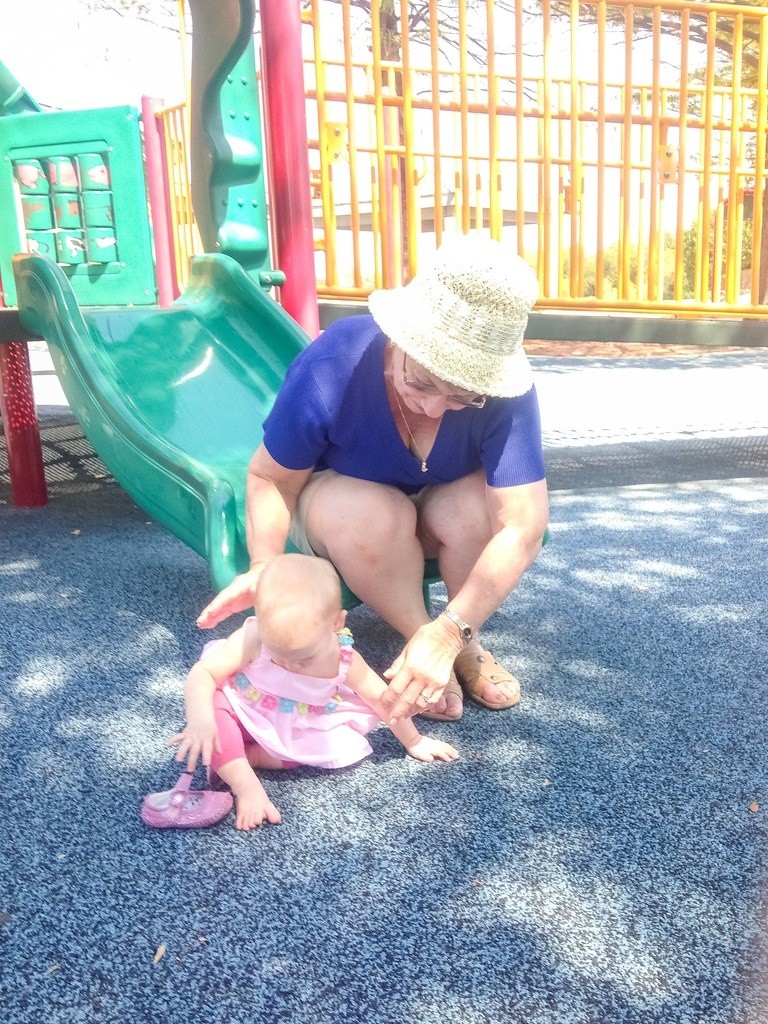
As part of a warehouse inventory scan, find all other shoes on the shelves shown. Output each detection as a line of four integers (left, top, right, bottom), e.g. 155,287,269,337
205,746,225,790
140,773,233,830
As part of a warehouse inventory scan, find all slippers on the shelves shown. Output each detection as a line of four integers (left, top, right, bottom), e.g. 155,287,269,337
418,668,463,720
455,649,520,710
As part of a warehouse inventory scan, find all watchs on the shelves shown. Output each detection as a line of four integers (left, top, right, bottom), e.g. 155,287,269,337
442,611,473,642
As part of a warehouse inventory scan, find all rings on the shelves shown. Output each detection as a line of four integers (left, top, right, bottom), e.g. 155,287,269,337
420,693,429,702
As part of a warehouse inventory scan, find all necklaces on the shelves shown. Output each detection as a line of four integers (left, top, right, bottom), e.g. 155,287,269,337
390,378,443,473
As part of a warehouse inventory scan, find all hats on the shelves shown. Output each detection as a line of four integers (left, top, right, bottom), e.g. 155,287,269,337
366,236,534,398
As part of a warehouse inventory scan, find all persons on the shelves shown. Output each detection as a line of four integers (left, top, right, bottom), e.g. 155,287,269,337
167,555,460,830
195,235,550,724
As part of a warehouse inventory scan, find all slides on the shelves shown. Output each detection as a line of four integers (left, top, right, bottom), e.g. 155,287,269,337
9,250,556,623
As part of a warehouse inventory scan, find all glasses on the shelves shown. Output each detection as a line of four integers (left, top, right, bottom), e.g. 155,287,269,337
401,352,486,409
